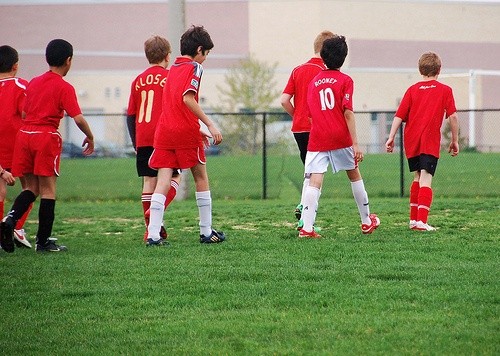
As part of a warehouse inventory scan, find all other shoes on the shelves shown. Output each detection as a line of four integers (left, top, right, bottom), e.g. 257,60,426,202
294,203,320,234
144,208,167,238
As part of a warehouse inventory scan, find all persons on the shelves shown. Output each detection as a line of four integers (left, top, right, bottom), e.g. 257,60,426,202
0,39,95,254
280,31,335,234
145,24,227,247
0,165,16,186
0,45,33,249
125,35,183,242
385,52,459,232
298,35,380,239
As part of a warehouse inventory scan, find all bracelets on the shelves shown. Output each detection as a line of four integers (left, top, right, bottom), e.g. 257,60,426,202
0,169,5,177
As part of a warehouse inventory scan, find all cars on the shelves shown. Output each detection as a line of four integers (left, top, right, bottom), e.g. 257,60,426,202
203,136,222,155
59,141,136,159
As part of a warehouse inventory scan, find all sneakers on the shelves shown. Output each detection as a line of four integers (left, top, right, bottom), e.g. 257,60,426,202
35,240,69,254
145,238,170,247
299,228,322,239
361,213,380,235
200,227,226,243
13,229,32,248
0,215,16,254
408,220,436,232
